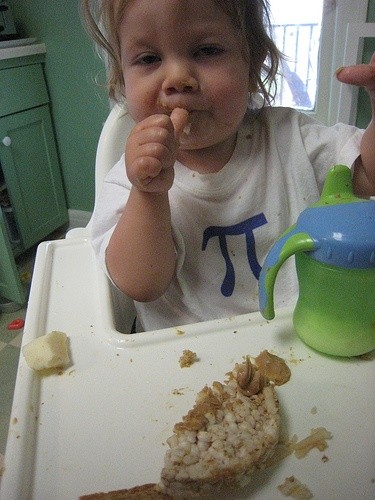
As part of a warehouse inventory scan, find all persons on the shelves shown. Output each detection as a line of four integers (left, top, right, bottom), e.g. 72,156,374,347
78,0,375,331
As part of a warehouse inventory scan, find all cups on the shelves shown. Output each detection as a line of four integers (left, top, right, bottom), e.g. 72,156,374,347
258,165,375,357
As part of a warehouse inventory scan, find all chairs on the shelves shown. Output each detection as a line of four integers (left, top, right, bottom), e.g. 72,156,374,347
92,93,270,333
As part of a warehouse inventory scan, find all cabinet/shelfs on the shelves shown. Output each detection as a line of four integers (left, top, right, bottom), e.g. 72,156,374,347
0,43,71,309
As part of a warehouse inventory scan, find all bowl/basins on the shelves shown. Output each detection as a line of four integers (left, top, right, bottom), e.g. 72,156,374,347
0,287,28,313
